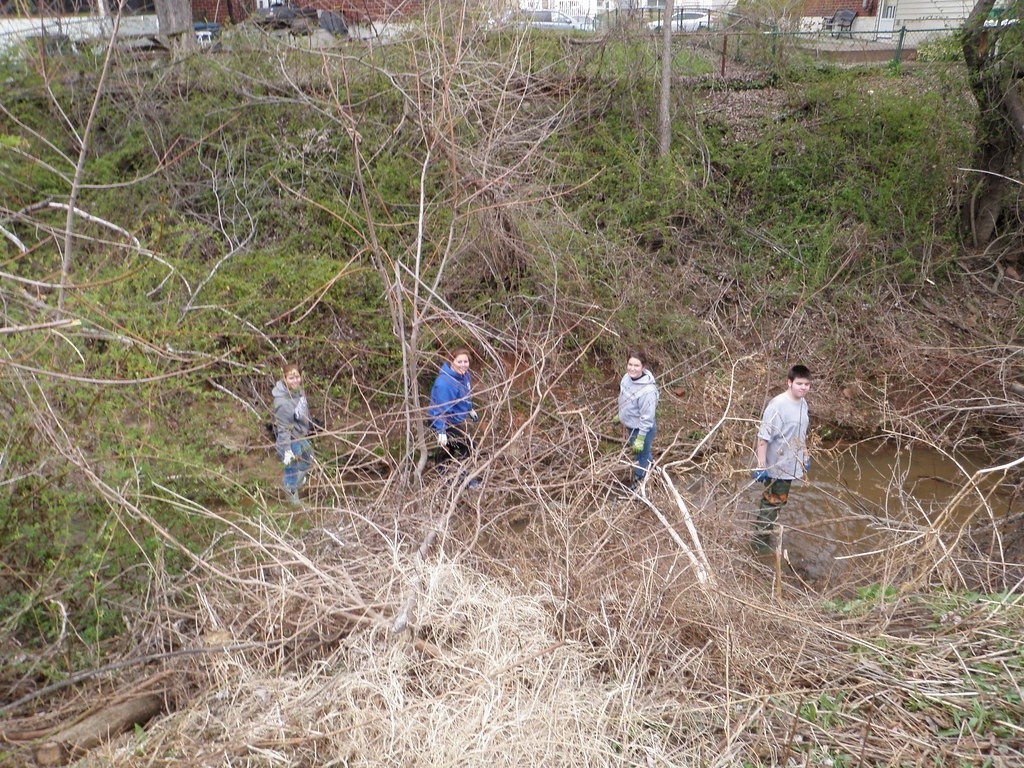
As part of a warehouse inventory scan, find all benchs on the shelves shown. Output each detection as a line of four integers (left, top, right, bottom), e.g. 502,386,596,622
818,9,858,41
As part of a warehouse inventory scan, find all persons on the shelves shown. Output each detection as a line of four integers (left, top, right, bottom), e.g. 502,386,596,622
271,364,314,508
753,365,813,536
427,349,478,485
618,353,657,489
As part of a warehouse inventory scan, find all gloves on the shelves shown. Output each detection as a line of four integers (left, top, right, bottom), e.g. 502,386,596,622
751,464,768,483
612,412,621,426
283,449,295,466
803,456,810,473
632,434,646,454
469,409,478,422
437,433,448,447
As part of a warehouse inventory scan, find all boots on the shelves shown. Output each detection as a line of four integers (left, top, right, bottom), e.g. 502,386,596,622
750,501,777,551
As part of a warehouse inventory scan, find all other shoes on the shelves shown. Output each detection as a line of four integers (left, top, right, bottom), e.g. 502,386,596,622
287,489,300,506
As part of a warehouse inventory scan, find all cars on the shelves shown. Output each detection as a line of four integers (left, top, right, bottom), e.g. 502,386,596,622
644,12,713,32
480,9,580,31
196,31,214,45
555,15,599,32
